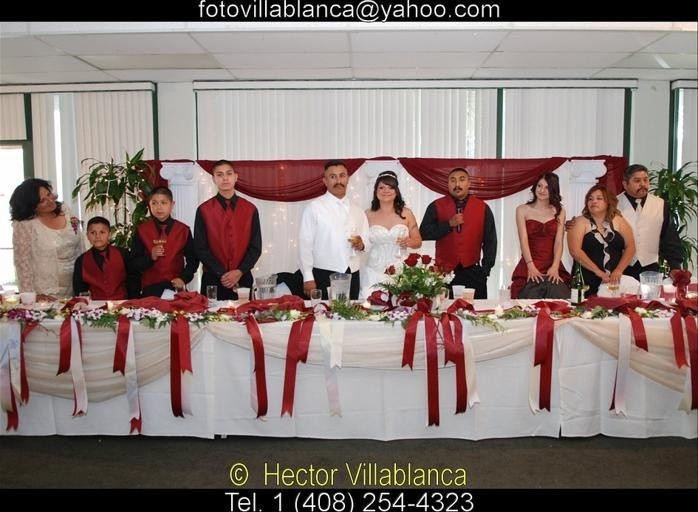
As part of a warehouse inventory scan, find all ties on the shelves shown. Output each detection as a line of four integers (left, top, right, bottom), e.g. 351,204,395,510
635,199,642,215
225,200,233,214
99,252,109,271
160,225,168,241
338,202,348,217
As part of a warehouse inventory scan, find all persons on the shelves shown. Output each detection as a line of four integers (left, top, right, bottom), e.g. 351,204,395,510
567,185,635,299
73,217,132,300
565,165,683,282
365,171,422,297
511,173,571,299
297,161,369,300
419,168,497,299
9,178,84,302
132,186,199,300
194,160,262,300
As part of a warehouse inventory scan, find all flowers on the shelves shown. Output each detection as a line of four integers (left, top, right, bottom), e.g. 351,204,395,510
403,253,455,296
370,265,414,297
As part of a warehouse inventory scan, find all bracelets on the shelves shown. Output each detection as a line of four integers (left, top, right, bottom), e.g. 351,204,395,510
525,260,534,264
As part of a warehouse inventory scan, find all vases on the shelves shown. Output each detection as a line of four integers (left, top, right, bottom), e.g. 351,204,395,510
400,296,416,307
417,295,433,314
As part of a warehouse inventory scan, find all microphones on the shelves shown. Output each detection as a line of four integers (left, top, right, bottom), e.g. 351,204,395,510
456,200,466,234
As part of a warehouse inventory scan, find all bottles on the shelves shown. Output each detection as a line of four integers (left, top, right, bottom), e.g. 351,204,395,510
571,254,587,305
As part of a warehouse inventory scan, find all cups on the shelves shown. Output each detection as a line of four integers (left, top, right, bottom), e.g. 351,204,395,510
252,276,278,300
207,286,217,305
452,285,465,301
464,288,475,304
20,291,37,305
330,271,352,305
238,288,250,308
311,288,322,307
3,292,21,305
607,277,621,295
639,270,664,303
